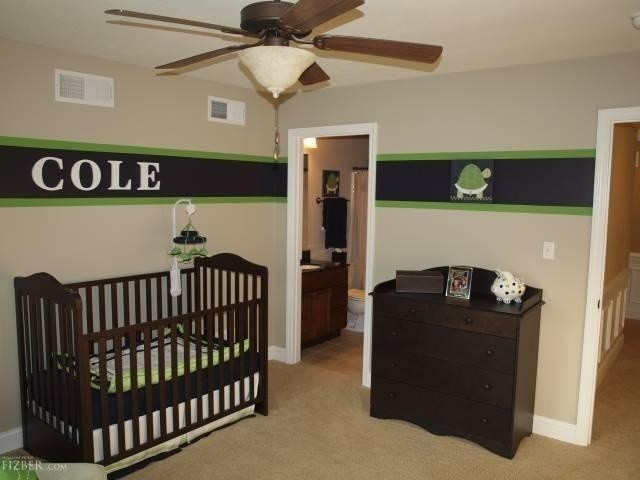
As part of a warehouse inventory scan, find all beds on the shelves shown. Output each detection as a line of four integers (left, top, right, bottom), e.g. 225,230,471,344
14,252,269,479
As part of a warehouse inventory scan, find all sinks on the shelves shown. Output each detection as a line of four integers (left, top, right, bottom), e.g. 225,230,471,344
301,264,320,270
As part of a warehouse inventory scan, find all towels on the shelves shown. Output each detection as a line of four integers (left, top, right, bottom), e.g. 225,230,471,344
322,197,347,253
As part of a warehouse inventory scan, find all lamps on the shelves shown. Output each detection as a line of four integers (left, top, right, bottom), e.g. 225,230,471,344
238,45,317,161
304,137,318,150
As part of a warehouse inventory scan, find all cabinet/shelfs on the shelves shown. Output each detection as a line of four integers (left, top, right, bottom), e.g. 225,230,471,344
301,265,348,350
370,267,546,460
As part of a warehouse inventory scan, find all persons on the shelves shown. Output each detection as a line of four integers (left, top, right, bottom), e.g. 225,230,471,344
451,270,467,292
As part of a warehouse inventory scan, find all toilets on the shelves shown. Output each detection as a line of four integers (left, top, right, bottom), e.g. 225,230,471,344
346,262,365,332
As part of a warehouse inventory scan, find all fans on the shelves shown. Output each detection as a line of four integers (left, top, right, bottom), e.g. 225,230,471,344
102,0,443,86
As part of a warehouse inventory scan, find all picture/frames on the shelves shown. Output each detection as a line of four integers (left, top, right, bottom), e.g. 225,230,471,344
445,267,474,299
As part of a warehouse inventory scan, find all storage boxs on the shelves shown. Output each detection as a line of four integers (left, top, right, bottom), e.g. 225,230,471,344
395,270,443,294
